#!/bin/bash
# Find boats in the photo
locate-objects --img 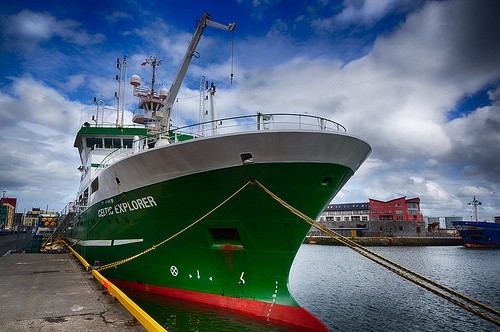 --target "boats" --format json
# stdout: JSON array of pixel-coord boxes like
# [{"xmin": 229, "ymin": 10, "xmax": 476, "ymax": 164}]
[
  {"xmin": 40, "ymin": 12, "xmax": 373, "ymax": 332},
  {"xmin": 448, "ymin": 220, "xmax": 499, "ymax": 248}
]
